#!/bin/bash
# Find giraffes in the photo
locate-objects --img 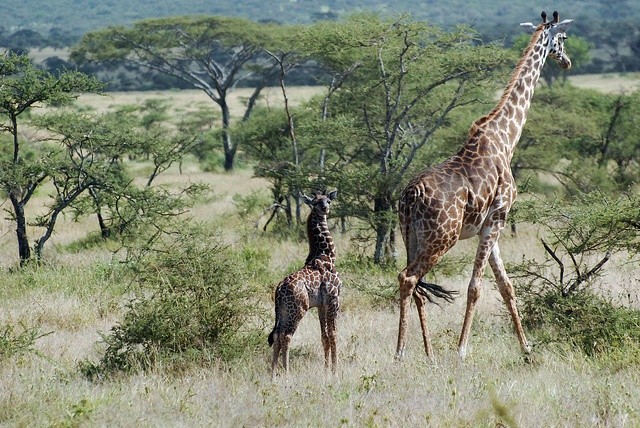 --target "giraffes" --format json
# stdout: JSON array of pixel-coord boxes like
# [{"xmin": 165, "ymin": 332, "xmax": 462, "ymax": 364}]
[
  {"xmin": 268, "ymin": 189, "xmax": 342, "ymax": 382},
  {"xmin": 395, "ymin": 11, "xmax": 574, "ymax": 363}
]
